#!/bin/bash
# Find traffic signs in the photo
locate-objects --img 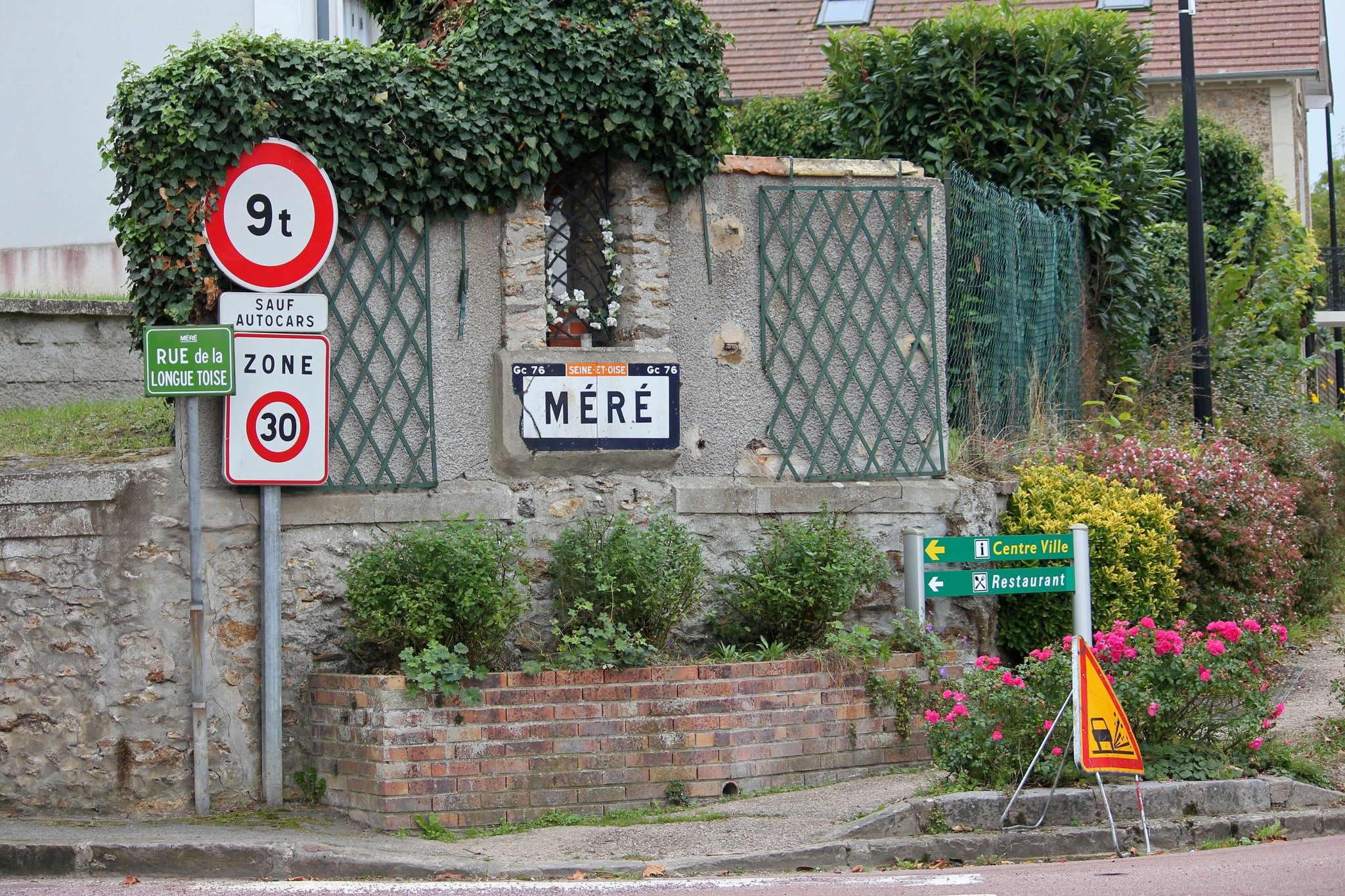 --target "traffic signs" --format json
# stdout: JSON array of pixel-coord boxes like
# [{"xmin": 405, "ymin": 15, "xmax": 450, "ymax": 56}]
[{"xmin": 922, "ymin": 534, "xmax": 1073, "ymax": 598}]
